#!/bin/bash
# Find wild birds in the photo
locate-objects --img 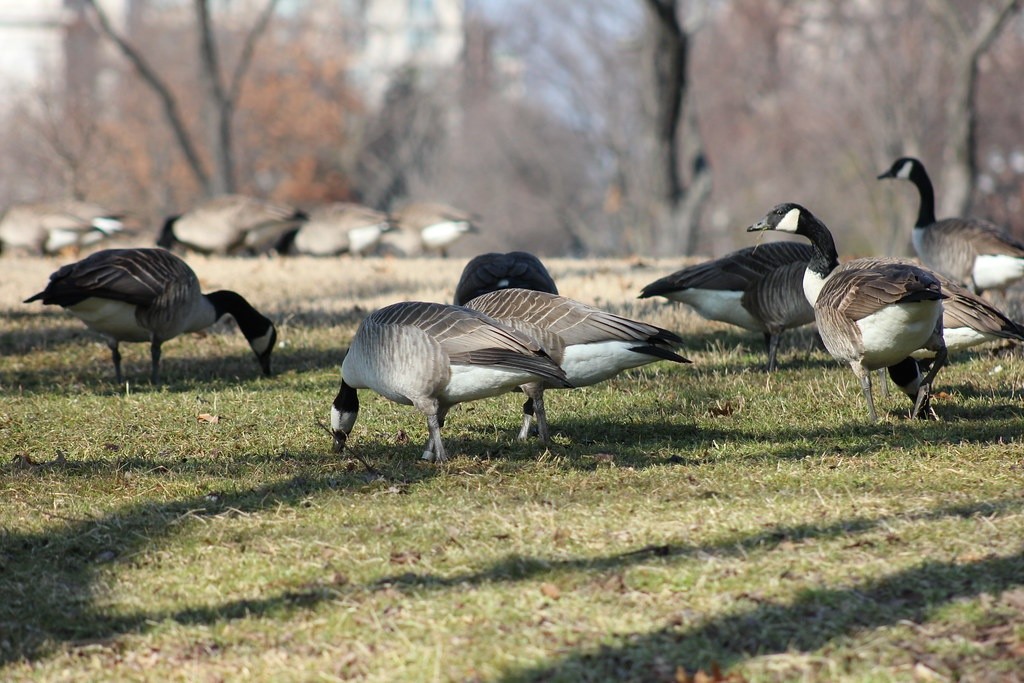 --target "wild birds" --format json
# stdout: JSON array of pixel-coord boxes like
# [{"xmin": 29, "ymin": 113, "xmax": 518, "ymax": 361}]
[
  {"xmin": 0, "ymin": 193, "xmax": 484, "ymax": 260},
  {"xmin": 329, "ymin": 249, "xmax": 694, "ymax": 464},
  {"xmin": 637, "ymin": 155, "xmax": 1024, "ymax": 424},
  {"xmin": 22, "ymin": 246, "xmax": 277, "ymax": 393}
]
